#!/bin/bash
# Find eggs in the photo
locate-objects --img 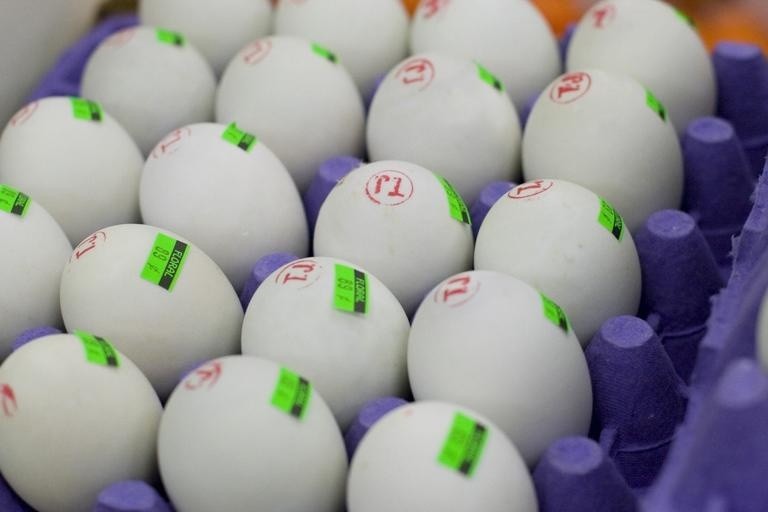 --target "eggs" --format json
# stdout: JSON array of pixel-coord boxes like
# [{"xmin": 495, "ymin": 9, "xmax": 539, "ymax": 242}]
[{"xmin": 0, "ymin": 0, "xmax": 717, "ymax": 512}]
